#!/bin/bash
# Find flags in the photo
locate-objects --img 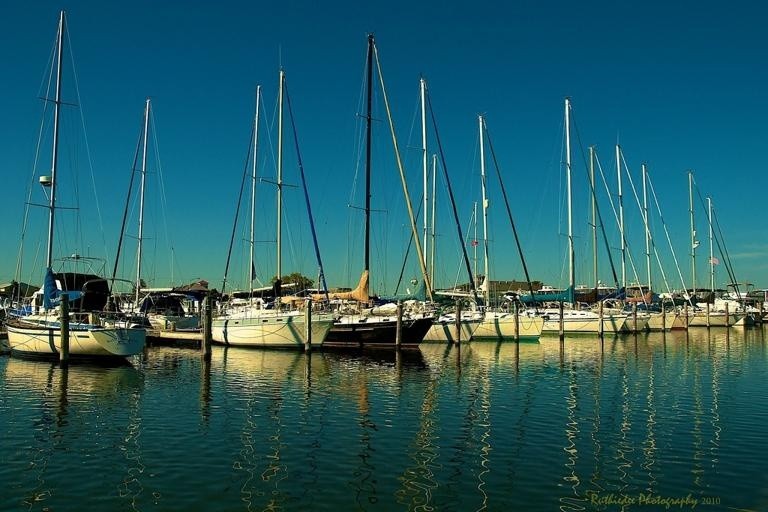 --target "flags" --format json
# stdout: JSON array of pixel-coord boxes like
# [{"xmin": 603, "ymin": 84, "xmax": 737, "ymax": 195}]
[{"xmin": 709, "ymin": 256, "xmax": 719, "ymax": 265}]
